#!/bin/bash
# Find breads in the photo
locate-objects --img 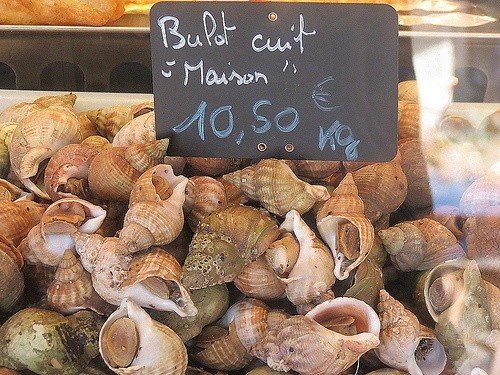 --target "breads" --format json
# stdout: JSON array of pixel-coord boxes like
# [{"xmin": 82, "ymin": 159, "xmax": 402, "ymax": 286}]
[{"xmin": 0, "ymin": 0, "xmax": 127, "ymax": 28}]
